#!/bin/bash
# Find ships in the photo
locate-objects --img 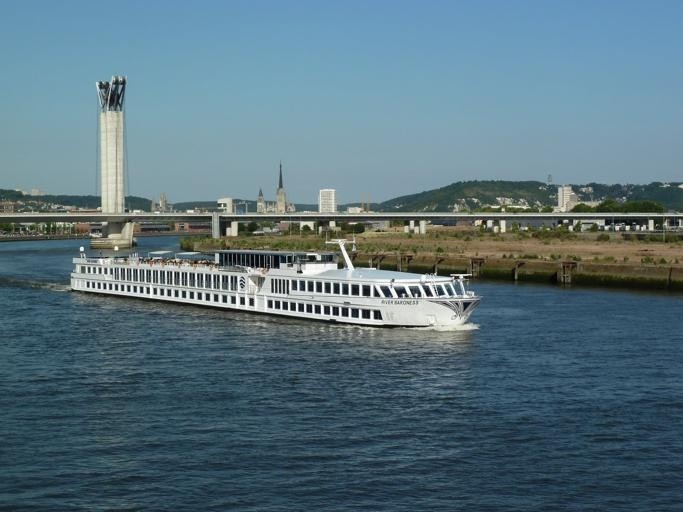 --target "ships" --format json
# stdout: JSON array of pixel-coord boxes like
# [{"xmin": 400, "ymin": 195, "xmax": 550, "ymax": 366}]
[{"xmin": 69, "ymin": 237, "xmax": 485, "ymax": 330}]
